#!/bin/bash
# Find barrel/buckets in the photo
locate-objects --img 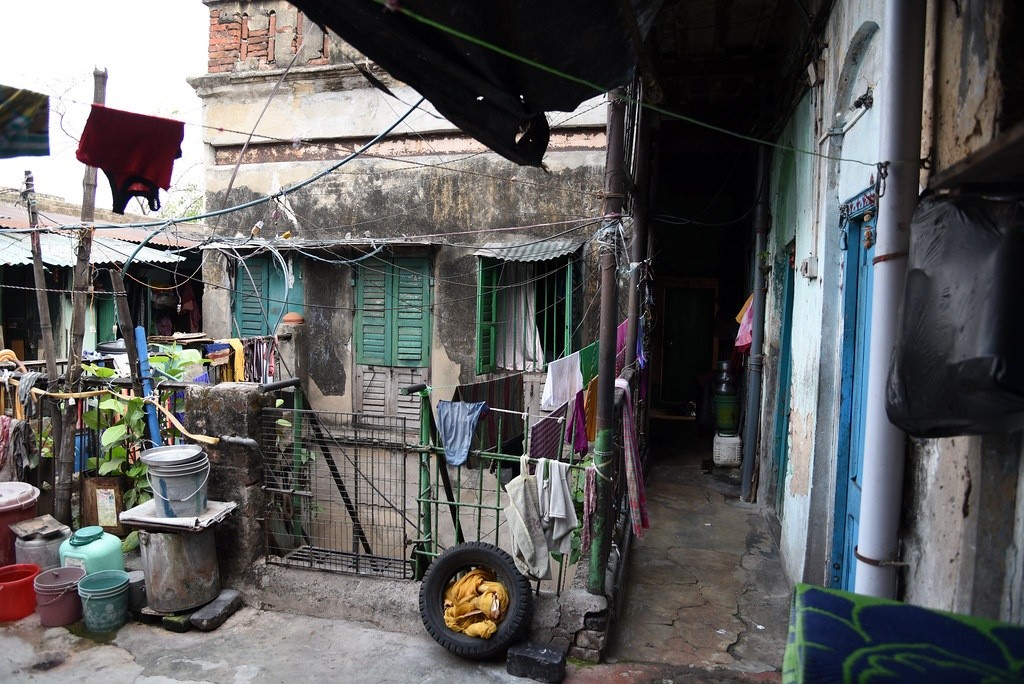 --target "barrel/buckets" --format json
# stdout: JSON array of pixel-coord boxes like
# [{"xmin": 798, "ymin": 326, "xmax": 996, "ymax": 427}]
[
  {"xmin": 146, "ymin": 451, "xmax": 211, "ymax": 519},
  {"xmin": 0, "ymin": 481, "xmax": 40, "ymax": 567},
  {"xmin": 0, "ymin": 564, "xmax": 40, "ymax": 622},
  {"xmin": 713, "ymin": 395, "xmax": 740, "ymax": 430},
  {"xmin": 15, "ymin": 526, "xmax": 73, "ymax": 574},
  {"xmin": 59, "ymin": 526, "xmax": 123, "ymax": 574},
  {"xmin": 78, "ymin": 570, "xmax": 130, "ymax": 633},
  {"xmin": 33, "ymin": 566, "xmax": 87, "ymax": 625}
]
